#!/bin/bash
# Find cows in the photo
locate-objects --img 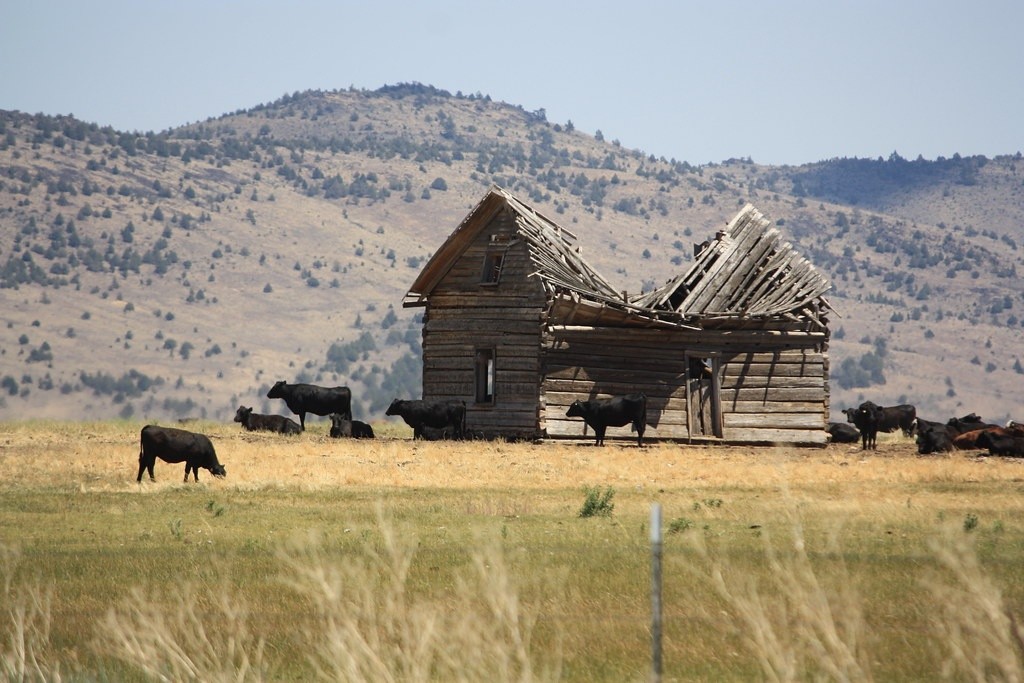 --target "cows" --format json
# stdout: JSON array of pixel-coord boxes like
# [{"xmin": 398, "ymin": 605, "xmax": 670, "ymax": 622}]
[
  {"xmin": 328, "ymin": 413, "xmax": 375, "ymax": 439},
  {"xmin": 137, "ymin": 425, "xmax": 227, "ymax": 483},
  {"xmin": 267, "ymin": 381, "xmax": 352, "ymax": 431},
  {"xmin": 386, "ymin": 398, "xmax": 467, "ymax": 441},
  {"xmin": 842, "ymin": 404, "xmax": 916, "ymax": 441},
  {"xmin": 828, "ymin": 422, "xmax": 860, "ymax": 443},
  {"xmin": 859, "ymin": 401, "xmax": 885, "ymax": 450},
  {"xmin": 912, "ymin": 413, "xmax": 1024, "ymax": 458},
  {"xmin": 566, "ymin": 394, "xmax": 647, "ymax": 448},
  {"xmin": 234, "ymin": 405, "xmax": 303, "ymax": 436}
]
